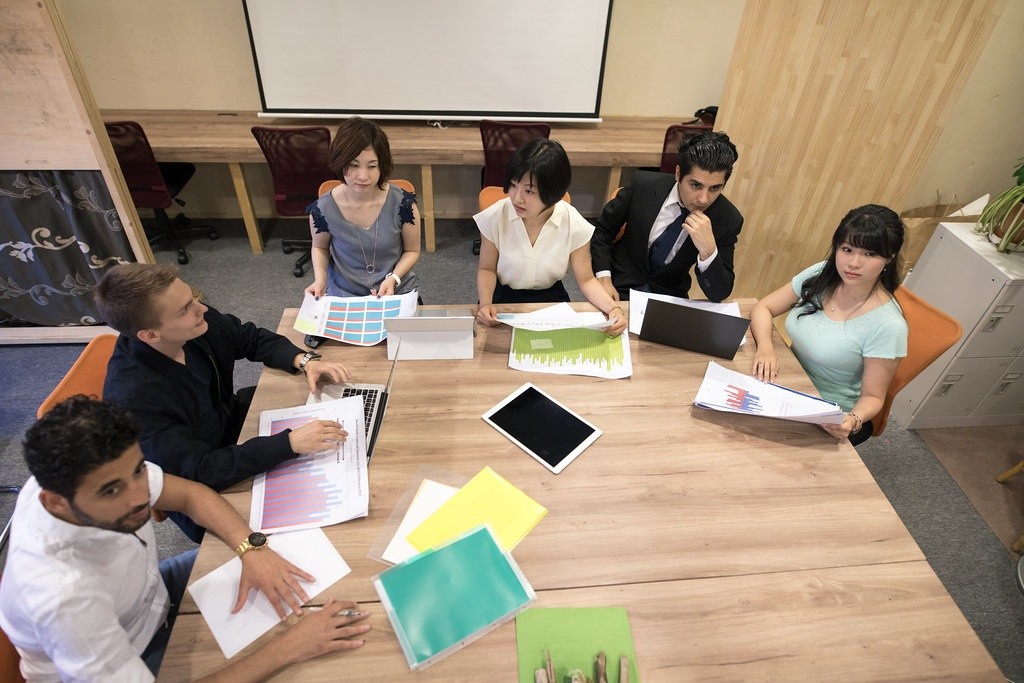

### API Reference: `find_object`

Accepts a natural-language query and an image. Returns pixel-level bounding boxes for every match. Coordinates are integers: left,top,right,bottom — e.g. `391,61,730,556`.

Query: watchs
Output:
386,272,401,288
299,350,320,376
236,532,268,558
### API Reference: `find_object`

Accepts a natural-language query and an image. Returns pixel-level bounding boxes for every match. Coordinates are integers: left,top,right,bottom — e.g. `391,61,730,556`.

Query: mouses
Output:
304,334,327,348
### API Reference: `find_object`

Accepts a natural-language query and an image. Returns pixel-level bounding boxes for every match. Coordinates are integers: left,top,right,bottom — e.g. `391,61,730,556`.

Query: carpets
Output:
917,422,1024,563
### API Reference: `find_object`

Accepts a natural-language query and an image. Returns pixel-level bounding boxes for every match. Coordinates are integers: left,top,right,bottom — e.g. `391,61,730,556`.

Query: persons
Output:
95,262,350,544
749,203,908,440
473,137,627,336
591,131,743,301
0,393,370,683
305,117,421,305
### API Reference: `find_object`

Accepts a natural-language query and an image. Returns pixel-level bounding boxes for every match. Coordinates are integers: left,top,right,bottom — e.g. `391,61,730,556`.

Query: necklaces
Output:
343,184,379,273
831,285,874,310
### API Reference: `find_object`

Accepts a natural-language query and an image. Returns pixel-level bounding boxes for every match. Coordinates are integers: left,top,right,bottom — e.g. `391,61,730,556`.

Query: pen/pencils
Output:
308,606,361,616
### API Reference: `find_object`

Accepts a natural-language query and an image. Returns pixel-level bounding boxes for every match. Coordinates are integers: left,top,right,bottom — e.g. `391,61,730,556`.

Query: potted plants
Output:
974,154,1024,254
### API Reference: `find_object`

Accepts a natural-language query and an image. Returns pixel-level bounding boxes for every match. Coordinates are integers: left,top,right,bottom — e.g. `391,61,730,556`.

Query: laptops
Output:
384,308,477,338
307,337,402,467
638,298,752,361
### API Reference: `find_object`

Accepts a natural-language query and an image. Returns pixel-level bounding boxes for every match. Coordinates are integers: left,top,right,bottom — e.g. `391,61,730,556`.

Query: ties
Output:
648,202,690,274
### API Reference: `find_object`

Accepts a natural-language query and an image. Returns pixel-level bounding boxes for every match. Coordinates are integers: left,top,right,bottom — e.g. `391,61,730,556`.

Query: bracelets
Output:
607,305,623,315
848,412,862,434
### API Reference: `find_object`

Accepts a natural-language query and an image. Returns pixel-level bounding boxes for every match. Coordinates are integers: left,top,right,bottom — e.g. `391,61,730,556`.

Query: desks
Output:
154,298,1015,683
97,108,702,255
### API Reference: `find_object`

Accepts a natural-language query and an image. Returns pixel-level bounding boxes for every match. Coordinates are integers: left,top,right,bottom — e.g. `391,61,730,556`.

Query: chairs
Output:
659,124,717,175
847,283,962,443
477,186,572,212
103,120,220,266
470,120,551,255
36,333,118,422
251,124,340,279
319,178,415,199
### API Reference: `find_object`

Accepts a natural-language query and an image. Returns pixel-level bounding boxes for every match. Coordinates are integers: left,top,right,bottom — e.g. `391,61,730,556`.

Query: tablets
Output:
481,381,602,475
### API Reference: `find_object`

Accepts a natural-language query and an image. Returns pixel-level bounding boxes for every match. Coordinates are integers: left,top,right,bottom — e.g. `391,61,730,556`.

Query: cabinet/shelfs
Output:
890,223,1024,431
